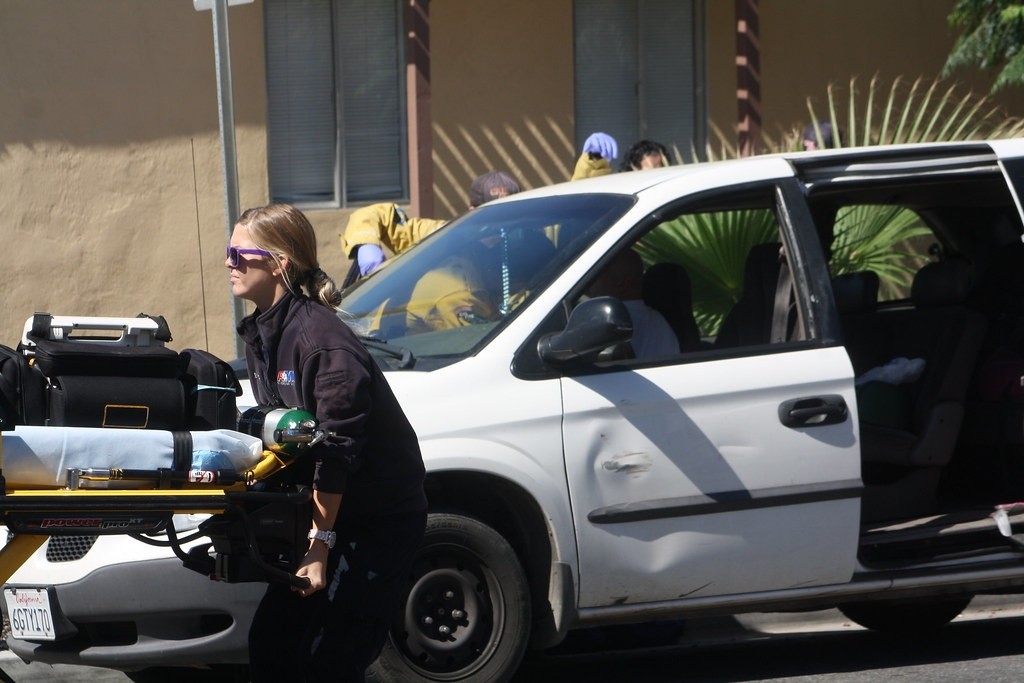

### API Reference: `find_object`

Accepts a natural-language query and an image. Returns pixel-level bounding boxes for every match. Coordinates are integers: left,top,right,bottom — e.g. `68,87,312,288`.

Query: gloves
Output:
357,245,384,276
584,132,618,163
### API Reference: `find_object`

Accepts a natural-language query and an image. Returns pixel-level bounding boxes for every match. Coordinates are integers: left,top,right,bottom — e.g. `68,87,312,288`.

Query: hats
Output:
470,171,521,207
803,120,843,149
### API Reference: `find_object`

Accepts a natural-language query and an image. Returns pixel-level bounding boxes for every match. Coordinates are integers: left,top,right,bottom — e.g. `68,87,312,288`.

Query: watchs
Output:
308,529,336,548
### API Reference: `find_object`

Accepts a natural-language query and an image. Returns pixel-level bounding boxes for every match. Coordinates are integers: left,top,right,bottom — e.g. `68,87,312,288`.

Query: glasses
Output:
227,247,274,268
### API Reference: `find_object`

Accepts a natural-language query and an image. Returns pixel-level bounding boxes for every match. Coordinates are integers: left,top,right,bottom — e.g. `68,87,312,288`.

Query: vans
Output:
227,138,1024,683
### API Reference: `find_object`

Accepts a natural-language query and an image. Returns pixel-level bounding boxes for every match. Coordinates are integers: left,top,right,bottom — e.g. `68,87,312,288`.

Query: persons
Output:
544,133,666,243
585,250,681,363
226,206,427,683
803,121,843,151
342,172,553,337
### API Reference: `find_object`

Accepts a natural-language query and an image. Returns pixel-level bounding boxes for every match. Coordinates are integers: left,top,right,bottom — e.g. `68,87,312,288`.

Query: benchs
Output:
837,258,986,468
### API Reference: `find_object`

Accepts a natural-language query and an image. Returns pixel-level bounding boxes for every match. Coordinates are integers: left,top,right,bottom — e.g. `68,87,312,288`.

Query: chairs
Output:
639,263,702,355
711,242,798,349
